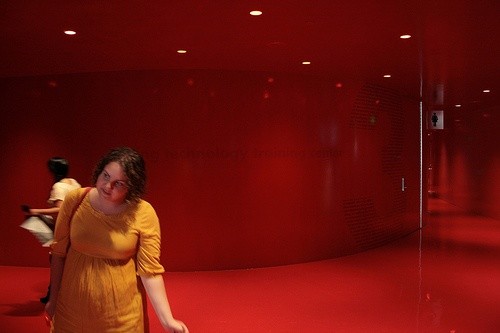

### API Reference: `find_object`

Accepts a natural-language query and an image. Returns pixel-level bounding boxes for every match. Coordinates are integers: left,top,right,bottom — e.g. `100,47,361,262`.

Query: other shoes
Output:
40,288,51,303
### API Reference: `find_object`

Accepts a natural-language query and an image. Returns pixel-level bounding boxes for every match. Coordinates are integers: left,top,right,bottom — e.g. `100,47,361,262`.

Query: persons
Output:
44,148,190,333
24,156,83,303
